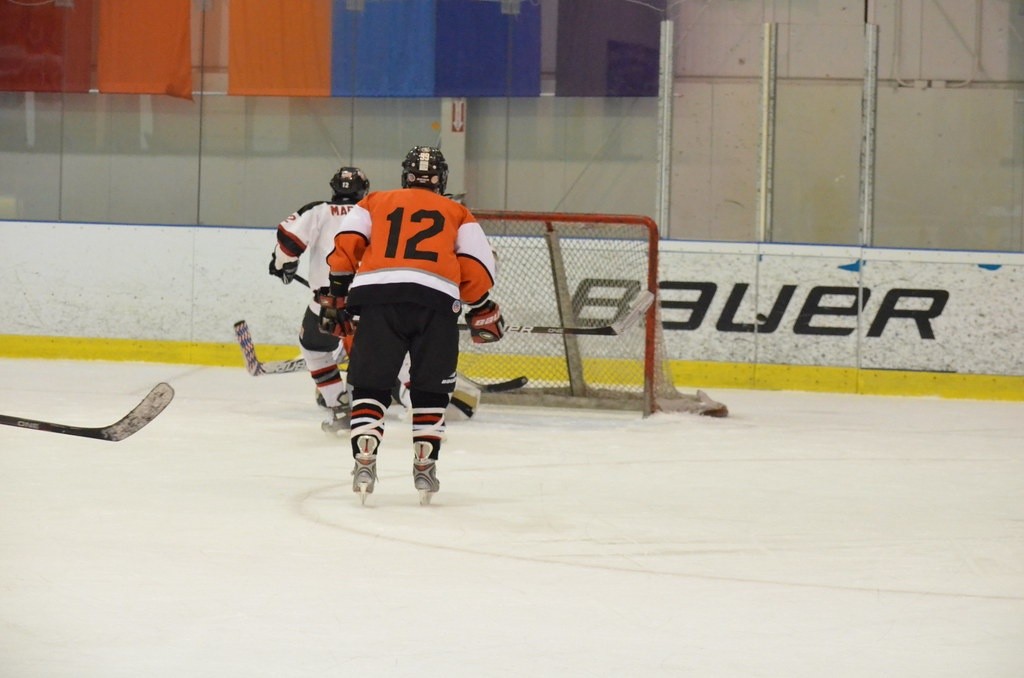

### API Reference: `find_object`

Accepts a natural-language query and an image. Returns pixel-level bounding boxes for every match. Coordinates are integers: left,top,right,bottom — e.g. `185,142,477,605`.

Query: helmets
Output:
402,146,449,194
329,167,369,200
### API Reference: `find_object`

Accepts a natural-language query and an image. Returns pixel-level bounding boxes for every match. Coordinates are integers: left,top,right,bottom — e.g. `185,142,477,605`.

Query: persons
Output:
326,146,506,506
270,166,411,436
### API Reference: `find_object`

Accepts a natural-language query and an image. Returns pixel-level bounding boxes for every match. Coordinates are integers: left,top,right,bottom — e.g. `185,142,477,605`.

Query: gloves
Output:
268,253,300,284
465,301,505,343
317,291,357,338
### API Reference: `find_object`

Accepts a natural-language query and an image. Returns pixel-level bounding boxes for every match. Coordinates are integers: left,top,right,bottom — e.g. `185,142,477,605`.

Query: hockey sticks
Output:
293,274,528,394
234,319,306,377
0,382,175,442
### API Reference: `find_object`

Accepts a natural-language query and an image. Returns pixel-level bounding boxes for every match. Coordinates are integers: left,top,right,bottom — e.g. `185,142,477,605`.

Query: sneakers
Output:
413,461,440,492
352,459,377,493
321,406,351,436
